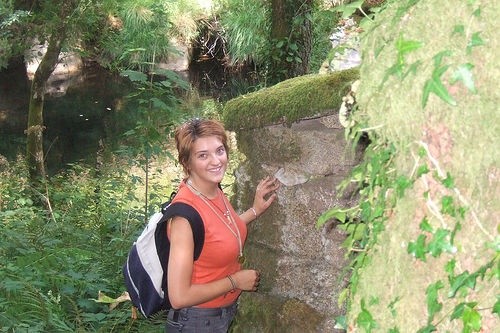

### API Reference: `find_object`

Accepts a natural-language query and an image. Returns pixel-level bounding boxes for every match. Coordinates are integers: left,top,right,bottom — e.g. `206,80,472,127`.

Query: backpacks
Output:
122,192,205,319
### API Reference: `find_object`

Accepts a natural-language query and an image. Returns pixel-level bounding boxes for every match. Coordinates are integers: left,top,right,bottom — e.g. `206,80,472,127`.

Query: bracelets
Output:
250,208,257,219
226,275,235,290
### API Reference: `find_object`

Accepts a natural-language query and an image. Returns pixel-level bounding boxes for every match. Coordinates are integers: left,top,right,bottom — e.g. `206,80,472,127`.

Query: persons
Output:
165,120,279,333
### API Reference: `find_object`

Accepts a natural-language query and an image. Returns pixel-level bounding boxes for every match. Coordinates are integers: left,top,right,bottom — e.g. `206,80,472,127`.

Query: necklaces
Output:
186,179,232,224
183,178,245,264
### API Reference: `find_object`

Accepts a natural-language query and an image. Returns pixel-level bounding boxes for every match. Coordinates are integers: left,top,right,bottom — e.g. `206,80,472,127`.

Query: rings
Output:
256,187,260,191
